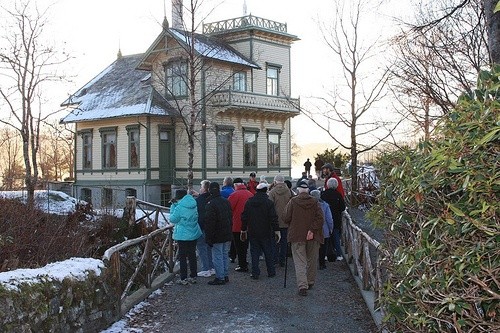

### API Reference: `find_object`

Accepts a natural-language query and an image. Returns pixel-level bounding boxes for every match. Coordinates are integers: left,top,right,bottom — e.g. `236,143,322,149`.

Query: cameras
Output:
168,199,176,204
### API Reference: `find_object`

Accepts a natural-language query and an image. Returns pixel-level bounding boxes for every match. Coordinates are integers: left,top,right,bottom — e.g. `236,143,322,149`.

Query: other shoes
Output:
324,256,328,261
187,277,197,284
178,278,188,286
250,274,257,279
309,284,314,290
209,268,215,275
208,278,226,285
225,276,229,282
228,257,235,263
320,264,326,269
337,256,343,261
299,288,307,296
197,270,211,277
235,266,248,272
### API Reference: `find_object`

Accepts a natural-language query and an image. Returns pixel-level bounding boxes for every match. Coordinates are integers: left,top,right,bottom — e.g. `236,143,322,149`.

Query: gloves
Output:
240,230,247,242
206,238,215,248
274,231,281,243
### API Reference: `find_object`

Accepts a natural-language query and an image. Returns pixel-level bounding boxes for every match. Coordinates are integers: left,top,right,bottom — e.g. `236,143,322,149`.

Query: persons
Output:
169,188,203,287
203,181,235,285
188,179,217,278
303,158,312,176
314,156,324,180
240,182,281,280
281,180,325,297
208,163,348,272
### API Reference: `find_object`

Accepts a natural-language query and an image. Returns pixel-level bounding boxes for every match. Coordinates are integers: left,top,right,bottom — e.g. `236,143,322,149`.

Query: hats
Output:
233,178,244,183
321,163,332,169
175,189,188,200
297,181,309,188
